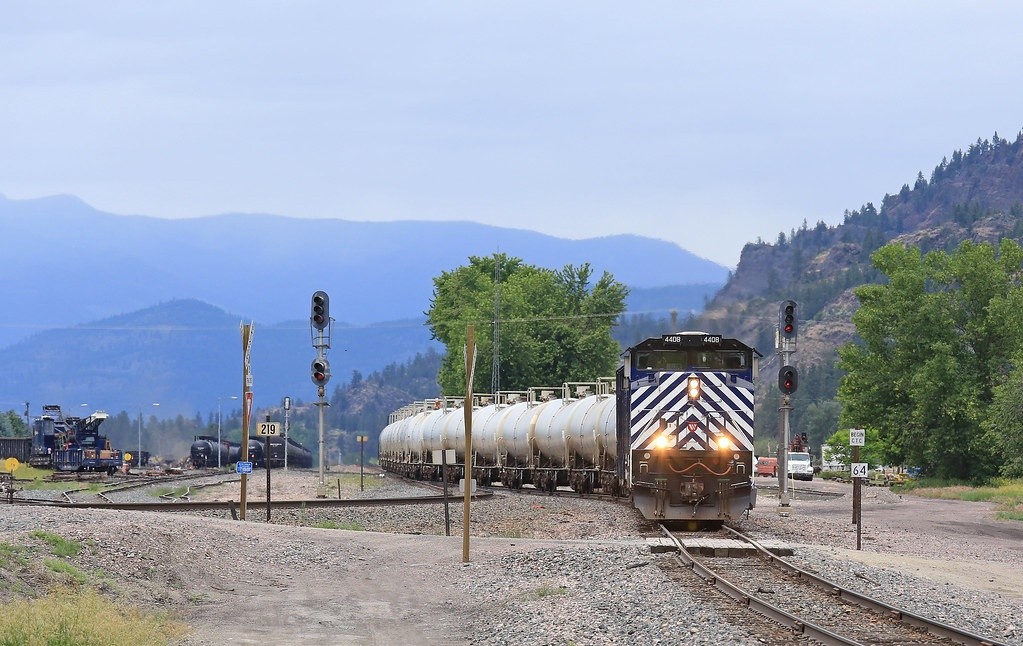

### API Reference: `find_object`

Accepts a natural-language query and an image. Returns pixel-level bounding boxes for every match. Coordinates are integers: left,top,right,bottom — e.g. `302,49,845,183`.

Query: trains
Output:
378,332,764,520
190,433,313,468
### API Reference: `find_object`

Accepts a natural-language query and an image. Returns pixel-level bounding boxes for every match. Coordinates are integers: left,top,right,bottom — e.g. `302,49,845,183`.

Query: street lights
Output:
138,403,159,469
218,397,239,470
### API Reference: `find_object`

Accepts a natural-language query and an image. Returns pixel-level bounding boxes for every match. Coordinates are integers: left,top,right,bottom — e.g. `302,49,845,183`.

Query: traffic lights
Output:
778,299,799,339
311,358,330,386
778,366,798,394
311,291,329,330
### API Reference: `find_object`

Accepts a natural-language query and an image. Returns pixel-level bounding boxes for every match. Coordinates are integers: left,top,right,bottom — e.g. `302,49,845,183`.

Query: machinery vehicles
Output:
28,402,123,475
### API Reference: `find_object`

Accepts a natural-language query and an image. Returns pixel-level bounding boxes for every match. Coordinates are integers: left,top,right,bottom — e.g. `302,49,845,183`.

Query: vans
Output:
788,451,813,481
